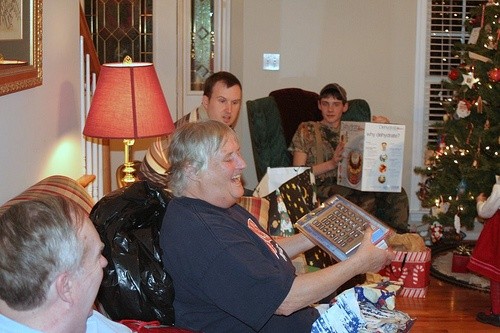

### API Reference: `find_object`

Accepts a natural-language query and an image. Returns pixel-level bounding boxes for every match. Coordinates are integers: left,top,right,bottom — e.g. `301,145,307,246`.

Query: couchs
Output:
0,175,95,220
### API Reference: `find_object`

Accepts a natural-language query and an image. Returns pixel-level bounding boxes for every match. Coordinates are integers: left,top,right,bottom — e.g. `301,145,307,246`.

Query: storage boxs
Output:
337,121,410,193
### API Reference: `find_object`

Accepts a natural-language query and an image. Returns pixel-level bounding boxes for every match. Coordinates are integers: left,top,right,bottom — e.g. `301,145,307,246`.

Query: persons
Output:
288,84,378,211
0,197,139,333
137,72,242,187
466,175,500,326
160,120,394,333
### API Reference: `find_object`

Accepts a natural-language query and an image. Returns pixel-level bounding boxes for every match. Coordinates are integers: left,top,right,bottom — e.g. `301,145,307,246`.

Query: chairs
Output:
247,88,411,268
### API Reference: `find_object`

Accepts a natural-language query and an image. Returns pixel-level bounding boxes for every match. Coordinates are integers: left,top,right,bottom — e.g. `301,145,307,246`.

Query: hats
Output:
320,83,347,102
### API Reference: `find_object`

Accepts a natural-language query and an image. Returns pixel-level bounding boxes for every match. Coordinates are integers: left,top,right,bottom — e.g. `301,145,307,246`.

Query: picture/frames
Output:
0,0,44,96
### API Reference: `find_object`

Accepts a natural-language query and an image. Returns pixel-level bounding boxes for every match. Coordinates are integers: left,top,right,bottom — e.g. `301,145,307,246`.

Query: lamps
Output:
83,56,176,186
